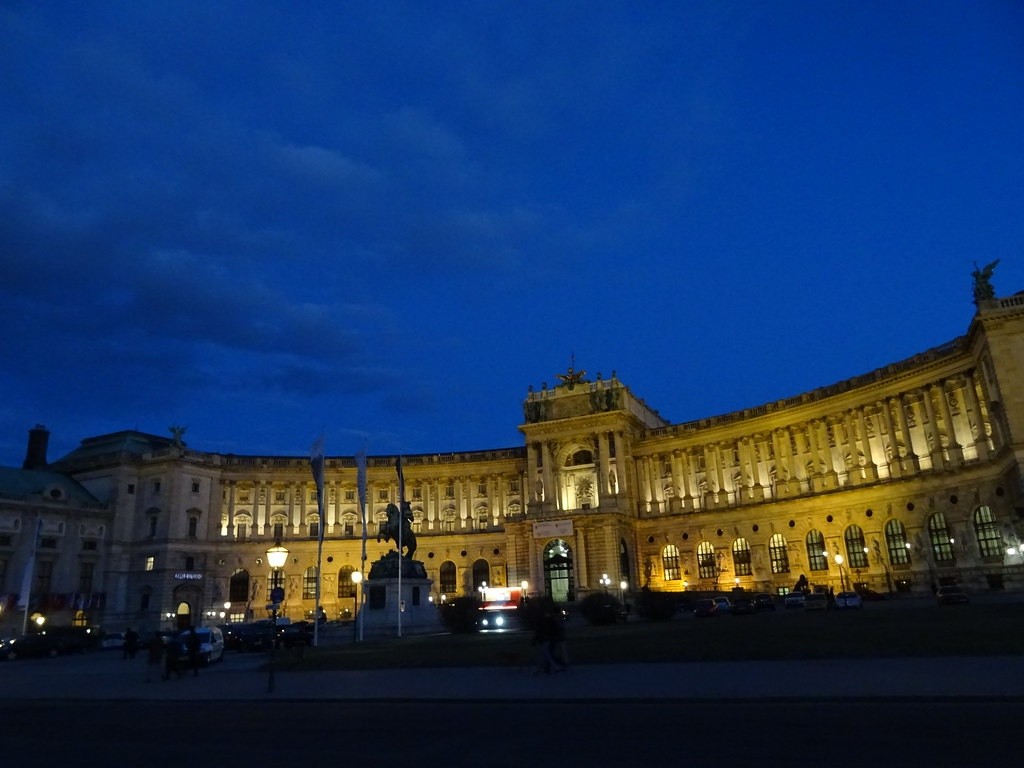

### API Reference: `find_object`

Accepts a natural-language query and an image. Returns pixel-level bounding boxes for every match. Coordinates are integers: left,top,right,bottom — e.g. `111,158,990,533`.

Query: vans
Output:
179,627,224,668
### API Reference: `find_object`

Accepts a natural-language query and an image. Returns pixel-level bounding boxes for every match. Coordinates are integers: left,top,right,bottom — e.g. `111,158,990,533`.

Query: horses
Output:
377,503,417,560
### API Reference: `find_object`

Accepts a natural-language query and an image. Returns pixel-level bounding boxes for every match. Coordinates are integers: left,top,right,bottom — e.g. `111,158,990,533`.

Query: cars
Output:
0,631,94,662
101,632,125,652
218,622,316,653
755,594,771,609
693,598,719,618
732,598,755,615
714,597,731,615
804,593,828,612
785,591,805,610
935,586,970,609
836,593,862,609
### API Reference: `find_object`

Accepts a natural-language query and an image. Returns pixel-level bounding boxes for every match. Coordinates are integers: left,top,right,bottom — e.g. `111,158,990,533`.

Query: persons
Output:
143,630,171,684
183,625,201,677
120,627,140,660
